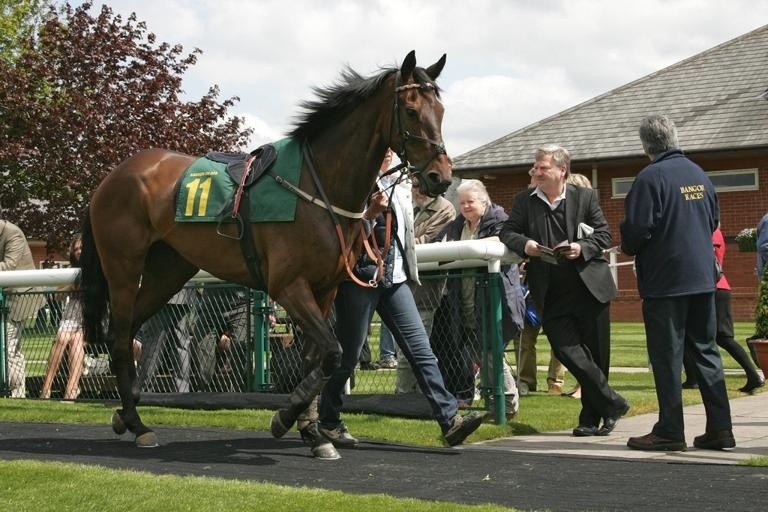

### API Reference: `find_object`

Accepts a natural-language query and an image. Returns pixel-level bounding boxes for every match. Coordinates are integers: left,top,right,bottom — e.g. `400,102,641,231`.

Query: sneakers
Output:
380,356,398,368
444,411,483,446
482,414,496,424
318,420,359,448
506,409,519,420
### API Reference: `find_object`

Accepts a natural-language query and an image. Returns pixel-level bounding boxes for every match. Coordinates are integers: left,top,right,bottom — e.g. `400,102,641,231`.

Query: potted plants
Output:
746,257,768,380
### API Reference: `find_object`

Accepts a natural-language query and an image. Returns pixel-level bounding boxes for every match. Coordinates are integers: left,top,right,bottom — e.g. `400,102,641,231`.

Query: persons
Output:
318,146,482,450
39,235,85,400
682,216,768,392
0,219,48,399
620,114,735,451
395,179,457,395
429,179,526,423
499,147,630,436
473,175,595,398
134,270,400,395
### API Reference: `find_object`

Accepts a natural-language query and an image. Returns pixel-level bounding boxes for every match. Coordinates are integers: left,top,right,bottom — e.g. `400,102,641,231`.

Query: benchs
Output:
55,335,294,397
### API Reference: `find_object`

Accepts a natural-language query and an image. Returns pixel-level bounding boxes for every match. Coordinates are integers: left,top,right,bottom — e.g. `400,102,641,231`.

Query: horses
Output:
78,50,453,460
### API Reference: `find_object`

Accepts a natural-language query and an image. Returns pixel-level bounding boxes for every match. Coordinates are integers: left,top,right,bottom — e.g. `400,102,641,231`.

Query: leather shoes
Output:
627,433,686,451
694,429,735,448
595,400,631,436
741,377,765,392
681,381,699,389
548,383,562,394
359,362,379,369
573,423,599,436
